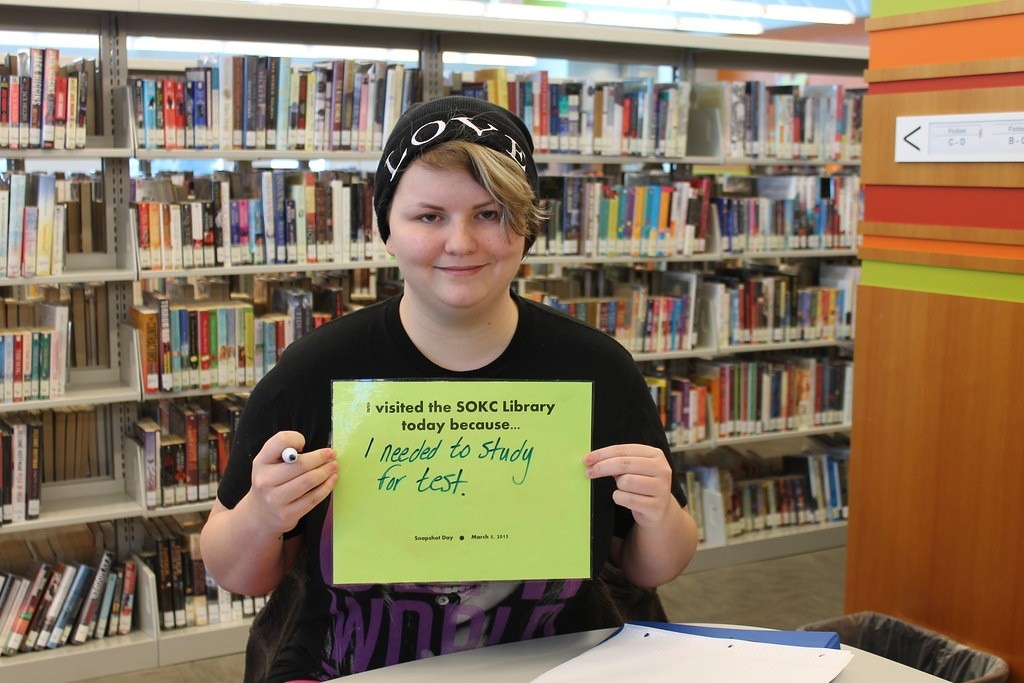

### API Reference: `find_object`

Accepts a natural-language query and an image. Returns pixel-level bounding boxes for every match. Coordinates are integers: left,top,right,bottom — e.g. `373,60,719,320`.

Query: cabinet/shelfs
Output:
712,31,869,575
431,13,712,579
0,2,158,682
115,0,430,669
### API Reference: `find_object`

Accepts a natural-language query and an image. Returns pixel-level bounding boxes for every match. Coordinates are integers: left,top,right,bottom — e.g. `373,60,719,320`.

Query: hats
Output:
374,96,540,252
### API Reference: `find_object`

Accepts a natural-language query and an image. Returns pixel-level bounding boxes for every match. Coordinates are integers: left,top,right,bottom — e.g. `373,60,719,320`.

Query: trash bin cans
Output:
794,610,1010,683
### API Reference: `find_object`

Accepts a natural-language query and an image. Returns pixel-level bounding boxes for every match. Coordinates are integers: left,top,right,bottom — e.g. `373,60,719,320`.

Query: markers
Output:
280,446,299,465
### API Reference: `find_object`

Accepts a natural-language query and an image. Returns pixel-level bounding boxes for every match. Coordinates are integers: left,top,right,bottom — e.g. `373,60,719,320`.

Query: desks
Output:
322,621,948,681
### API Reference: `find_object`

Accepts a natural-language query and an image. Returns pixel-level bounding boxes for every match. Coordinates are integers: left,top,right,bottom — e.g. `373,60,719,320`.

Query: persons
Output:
202,95,699,683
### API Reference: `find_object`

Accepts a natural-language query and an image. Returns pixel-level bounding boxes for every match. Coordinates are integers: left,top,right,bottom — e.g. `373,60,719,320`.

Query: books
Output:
1,428,848,668
0,163,867,279
0,258,861,407
0,347,854,531
0,39,876,158
